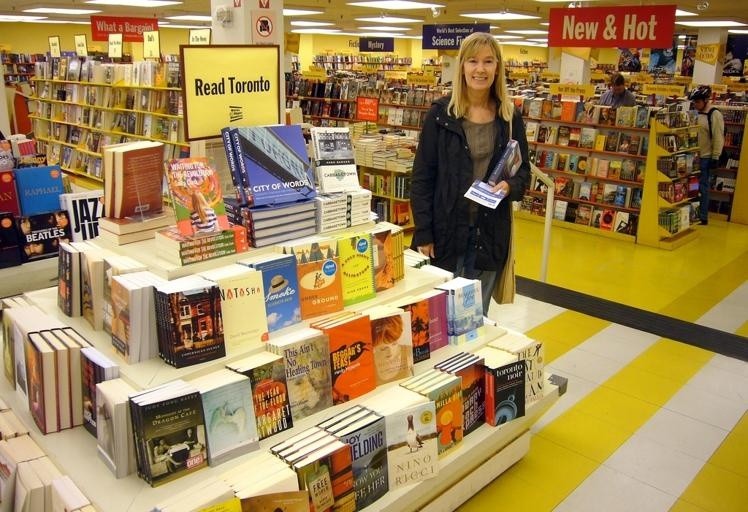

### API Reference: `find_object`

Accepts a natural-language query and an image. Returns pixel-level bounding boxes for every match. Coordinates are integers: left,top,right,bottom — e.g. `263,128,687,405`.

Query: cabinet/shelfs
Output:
422,59,443,83
285,72,448,143
637,102,748,222
508,96,650,244
302,144,417,232
0,65,33,141
0,52,133,114
313,52,412,78
27,57,190,206
637,119,704,251
589,64,615,83
503,61,548,87
0,182,91,298
1,221,567,511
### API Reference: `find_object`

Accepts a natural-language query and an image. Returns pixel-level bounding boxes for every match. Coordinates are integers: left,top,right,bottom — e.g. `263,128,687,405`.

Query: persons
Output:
183,428,197,451
154,438,182,473
599,74,636,108
17,210,69,258
371,316,414,385
687,86,724,224
412,299,428,320
410,33,532,318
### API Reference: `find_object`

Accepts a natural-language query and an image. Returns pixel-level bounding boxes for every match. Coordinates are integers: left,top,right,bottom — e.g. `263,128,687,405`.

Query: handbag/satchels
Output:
490,103,518,306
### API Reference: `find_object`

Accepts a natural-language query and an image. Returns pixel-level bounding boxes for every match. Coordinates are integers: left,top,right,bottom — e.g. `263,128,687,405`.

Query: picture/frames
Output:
178,44,280,141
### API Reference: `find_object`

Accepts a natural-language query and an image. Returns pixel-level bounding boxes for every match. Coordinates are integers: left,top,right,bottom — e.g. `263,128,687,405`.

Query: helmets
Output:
687,85,712,101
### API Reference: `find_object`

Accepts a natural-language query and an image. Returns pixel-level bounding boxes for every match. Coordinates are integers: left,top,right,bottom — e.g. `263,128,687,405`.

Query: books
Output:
0,133,105,269
27,62,191,181
0,397,98,512
357,351,488,490
344,121,415,226
98,125,373,268
128,350,295,488
153,222,406,369
55,241,166,365
656,104,690,235
0,294,135,480
266,277,486,422
287,56,441,138
154,331,545,510
510,98,649,235
152,406,389,512
